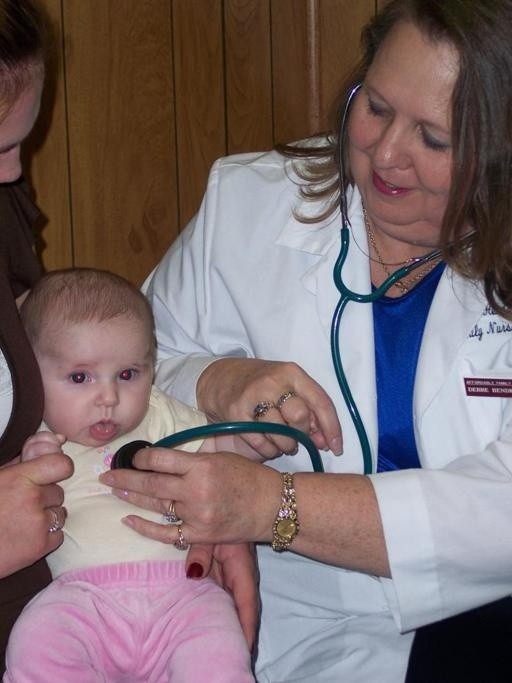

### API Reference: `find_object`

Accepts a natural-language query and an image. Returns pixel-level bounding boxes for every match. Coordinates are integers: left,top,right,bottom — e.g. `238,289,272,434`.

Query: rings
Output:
47,505,65,533
252,400,275,420
276,389,296,410
175,523,189,551
165,498,178,522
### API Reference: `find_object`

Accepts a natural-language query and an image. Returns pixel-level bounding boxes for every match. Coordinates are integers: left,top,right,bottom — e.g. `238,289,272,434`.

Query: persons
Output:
0,266,264,681
95,0,511,682
0,2,264,682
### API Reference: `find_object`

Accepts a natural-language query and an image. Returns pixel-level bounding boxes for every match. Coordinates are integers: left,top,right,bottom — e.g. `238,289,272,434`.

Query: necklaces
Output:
361,198,448,295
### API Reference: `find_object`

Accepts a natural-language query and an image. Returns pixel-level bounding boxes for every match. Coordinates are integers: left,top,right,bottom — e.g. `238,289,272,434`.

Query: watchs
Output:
270,469,301,553
